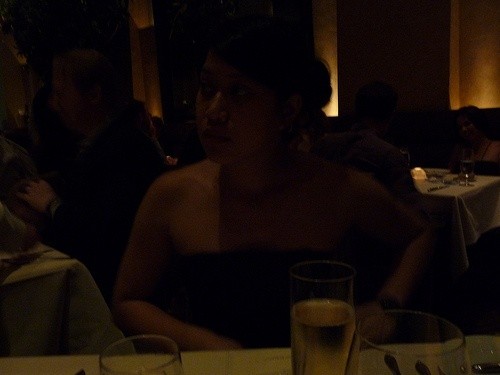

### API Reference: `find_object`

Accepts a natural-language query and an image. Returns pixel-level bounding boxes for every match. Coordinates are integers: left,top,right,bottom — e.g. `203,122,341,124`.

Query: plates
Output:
437,335,500,375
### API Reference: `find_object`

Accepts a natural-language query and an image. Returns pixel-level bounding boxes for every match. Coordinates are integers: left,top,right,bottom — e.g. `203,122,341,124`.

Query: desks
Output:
0,335,500,375
416,173,500,270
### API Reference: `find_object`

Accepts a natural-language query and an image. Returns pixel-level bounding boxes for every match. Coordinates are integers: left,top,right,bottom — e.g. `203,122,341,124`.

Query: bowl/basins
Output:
424,168,450,177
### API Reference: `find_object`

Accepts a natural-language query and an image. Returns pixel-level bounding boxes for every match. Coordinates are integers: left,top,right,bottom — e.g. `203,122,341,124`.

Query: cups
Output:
343,310,471,375
457,148,475,186
98,334,185,375
288,261,359,375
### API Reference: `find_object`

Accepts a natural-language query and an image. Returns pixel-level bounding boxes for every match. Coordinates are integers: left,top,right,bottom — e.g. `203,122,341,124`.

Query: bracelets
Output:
378,299,400,314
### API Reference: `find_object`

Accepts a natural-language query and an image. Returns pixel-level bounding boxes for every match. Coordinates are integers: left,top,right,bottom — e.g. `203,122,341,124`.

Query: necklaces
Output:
480,141,493,161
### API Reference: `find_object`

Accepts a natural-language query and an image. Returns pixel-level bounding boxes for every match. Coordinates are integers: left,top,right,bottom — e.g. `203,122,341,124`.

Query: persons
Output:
0,49,164,358
283,57,453,272
110,13,437,351
452,102,500,177
